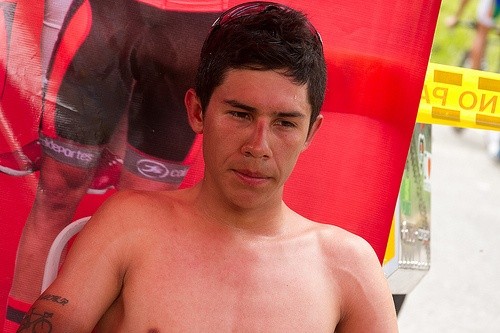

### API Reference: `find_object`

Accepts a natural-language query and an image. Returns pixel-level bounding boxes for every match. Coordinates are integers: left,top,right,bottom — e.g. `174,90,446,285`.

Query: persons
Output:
6,1,227,332
15,7,399,333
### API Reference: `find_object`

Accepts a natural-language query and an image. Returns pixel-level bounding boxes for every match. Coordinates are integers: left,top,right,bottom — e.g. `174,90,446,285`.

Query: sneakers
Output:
86,148,124,195
0,140,44,177
